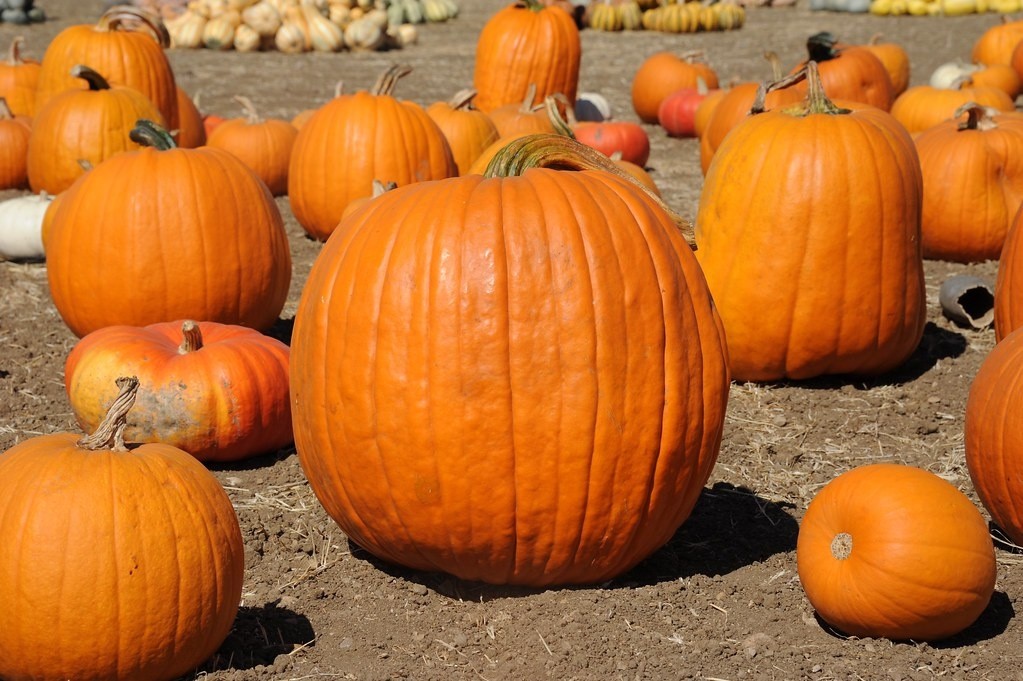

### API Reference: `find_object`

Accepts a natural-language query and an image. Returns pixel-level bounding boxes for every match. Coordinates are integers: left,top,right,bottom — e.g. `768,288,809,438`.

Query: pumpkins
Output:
796,465,996,644
0,0,1023,584
0,376,247,681
